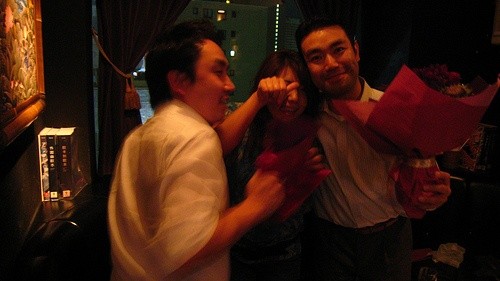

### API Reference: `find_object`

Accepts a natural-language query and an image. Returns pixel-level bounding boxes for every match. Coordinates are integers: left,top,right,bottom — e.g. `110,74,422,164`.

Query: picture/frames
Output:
0,0,48,150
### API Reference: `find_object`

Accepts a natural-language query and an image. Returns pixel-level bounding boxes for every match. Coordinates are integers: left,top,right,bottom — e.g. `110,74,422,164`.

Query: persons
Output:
295,14,451,281
212,49,330,281
106,18,289,281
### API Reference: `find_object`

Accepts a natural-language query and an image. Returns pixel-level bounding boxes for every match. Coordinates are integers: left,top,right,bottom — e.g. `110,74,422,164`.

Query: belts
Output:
355,216,400,236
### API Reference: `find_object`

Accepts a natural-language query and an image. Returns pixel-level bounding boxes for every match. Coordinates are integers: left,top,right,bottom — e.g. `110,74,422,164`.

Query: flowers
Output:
332,64,500,219
